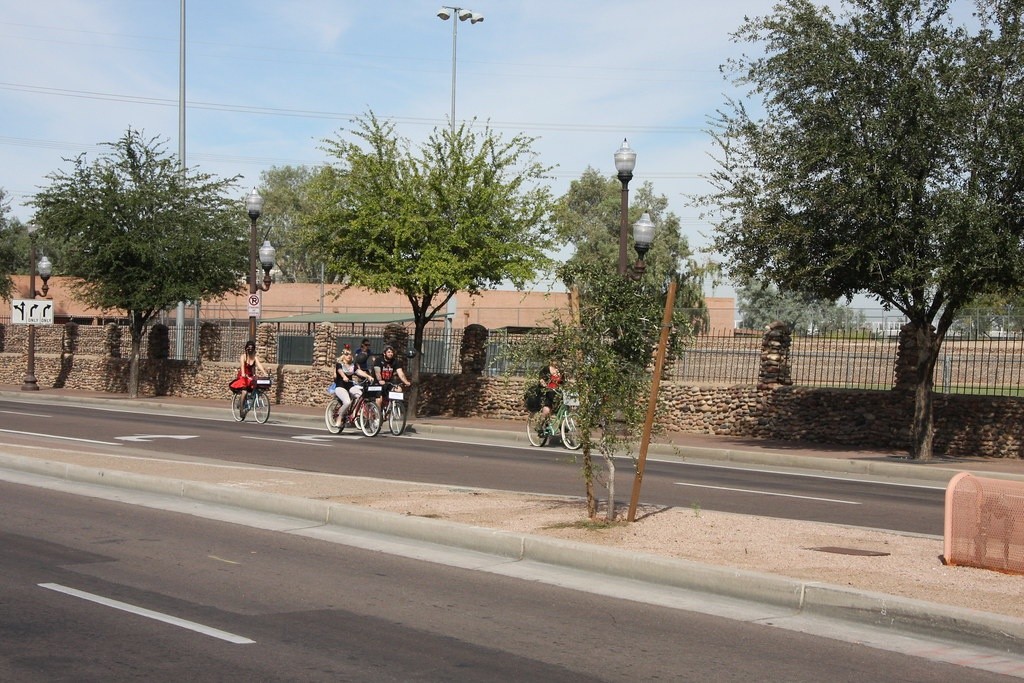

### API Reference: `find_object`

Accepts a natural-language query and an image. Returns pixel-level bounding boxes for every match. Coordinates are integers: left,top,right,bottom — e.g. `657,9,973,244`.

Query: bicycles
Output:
525,381,585,450
229,368,272,424
327,392,369,429
322,377,384,438
351,377,412,435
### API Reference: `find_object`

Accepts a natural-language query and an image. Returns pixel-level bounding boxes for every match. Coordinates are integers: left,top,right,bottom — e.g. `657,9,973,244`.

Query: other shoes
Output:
240,407,245,418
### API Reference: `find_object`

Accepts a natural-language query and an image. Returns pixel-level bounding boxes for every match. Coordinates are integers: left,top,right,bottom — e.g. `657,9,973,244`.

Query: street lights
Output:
436,5,484,183
16,220,54,393
230,186,278,342
611,136,656,286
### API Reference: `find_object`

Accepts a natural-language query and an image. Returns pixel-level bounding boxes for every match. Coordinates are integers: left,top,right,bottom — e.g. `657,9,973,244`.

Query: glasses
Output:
364,344,370,346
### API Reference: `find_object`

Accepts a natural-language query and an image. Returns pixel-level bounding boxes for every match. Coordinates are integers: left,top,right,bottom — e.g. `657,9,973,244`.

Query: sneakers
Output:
336,417,342,428
558,424,570,431
534,424,544,438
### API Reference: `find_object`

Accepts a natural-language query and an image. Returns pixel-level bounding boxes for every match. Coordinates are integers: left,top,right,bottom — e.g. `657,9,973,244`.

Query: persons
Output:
375,345,412,429
334,357,362,423
327,344,375,429
230,342,270,420
356,338,372,387
364,356,380,430
537,355,571,437
